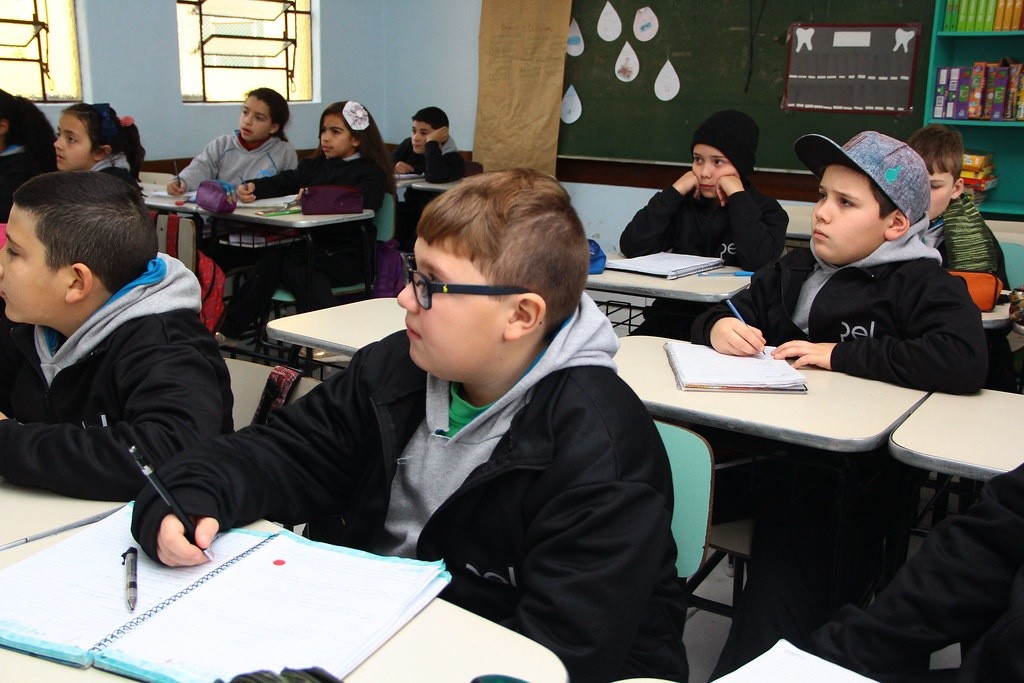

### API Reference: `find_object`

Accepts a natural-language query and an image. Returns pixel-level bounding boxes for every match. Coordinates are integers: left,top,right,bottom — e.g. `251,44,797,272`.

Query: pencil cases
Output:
303,184,364,213
194,178,237,215
950,270,1001,312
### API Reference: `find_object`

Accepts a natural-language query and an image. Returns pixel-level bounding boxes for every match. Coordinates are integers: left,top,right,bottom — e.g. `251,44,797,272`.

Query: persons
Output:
904,124,1015,395
217,100,397,362
619,110,789,342
0,88,58,224
705,130,987,683
0,170,236,503
168,87,298,272
54,102,146,203
130,168,690,683
392,105,467,270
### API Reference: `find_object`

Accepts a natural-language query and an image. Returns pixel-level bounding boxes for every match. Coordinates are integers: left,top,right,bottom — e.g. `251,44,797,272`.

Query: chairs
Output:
138,172,175,184
990,231,1024,290
251,194,395,363
220,355,321,431
648,415,755,627
155,214,195,273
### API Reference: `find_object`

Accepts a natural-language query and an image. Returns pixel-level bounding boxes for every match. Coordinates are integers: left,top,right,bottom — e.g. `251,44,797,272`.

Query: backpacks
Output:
149,210,225,334
366,239,406,300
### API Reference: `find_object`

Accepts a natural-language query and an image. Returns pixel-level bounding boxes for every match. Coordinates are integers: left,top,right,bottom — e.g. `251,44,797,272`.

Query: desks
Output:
5,205,1024,683
137,182,459,367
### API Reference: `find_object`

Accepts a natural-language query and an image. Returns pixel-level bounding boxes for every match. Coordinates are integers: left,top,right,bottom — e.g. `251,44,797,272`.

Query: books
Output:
603,252,725,281
150,191,198,204
0,499,452,683
236,194,299,210
663,339,809,395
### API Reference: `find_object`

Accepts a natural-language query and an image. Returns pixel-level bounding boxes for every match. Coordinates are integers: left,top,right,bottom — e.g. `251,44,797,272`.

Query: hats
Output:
794,131,932,224
689,110,758,190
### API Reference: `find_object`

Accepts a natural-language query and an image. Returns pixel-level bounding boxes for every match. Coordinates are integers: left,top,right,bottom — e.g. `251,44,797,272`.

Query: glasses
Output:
400,252,544,326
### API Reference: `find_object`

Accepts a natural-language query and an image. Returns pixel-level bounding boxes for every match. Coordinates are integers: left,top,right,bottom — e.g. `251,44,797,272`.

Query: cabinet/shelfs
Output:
923,0,1024,215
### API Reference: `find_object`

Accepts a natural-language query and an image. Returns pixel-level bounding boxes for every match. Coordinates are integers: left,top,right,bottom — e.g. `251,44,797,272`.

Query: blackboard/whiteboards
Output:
558,0,937,204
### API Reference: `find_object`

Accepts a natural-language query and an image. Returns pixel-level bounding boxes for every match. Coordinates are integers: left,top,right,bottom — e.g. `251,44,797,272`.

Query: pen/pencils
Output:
696,270,755,282
726,297,769,357
173,159,180,188
239,173,253,195
129,443,216,565
121,545,139,612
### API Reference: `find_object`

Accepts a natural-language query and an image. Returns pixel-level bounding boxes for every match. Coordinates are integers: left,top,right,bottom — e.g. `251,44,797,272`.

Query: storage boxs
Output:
960,149,1000,191
933,57,1024,121
